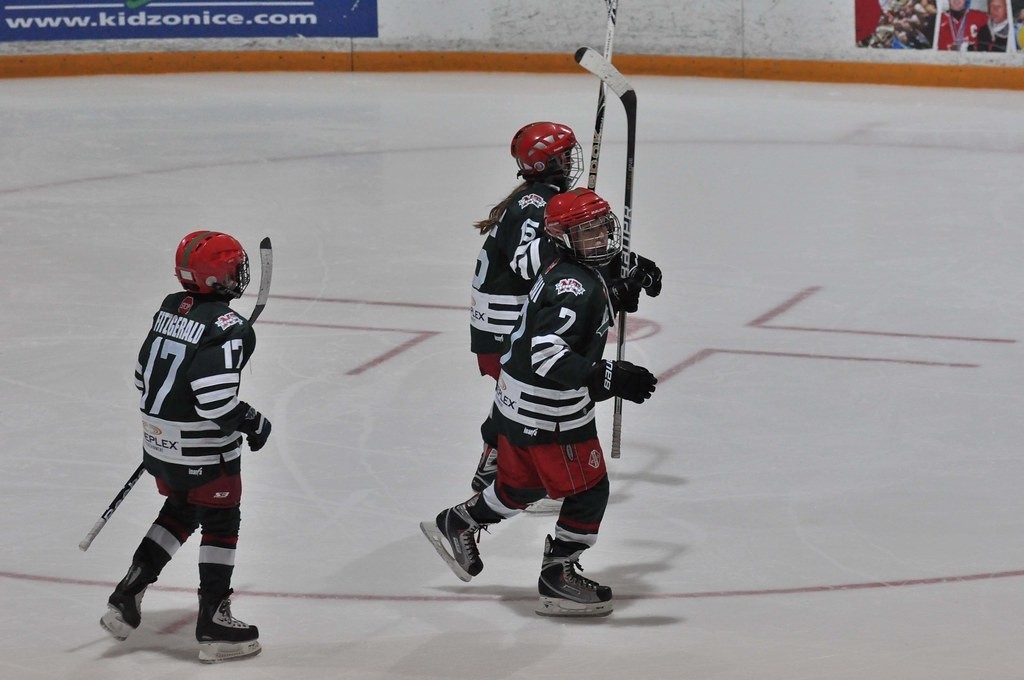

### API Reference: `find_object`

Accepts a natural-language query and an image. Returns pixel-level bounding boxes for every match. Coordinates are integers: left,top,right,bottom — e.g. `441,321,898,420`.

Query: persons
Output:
420,186,657,616
99,231,273,665
1013,8,1024,52
974,0,1009,52
855,0,937,49
939,0,989,52
471,122,662,515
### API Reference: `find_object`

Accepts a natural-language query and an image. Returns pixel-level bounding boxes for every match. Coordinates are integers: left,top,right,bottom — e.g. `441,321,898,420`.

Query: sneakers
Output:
534,533,614,618
100,561,150,643
467,443,500,493
194,587,263,664
419,490,503,583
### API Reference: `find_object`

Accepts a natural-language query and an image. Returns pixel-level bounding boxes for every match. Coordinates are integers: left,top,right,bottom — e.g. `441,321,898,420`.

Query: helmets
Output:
510,121,584,189
543,186,622,264
175,230,251,300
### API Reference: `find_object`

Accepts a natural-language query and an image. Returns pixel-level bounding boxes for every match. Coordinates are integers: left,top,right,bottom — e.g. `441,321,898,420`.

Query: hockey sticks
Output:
586,0,619,192
574,46,638,459
79,237,273,552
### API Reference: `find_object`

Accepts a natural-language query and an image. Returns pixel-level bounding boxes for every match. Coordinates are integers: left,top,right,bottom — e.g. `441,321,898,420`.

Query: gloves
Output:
604,276,643,314
235,403,272,452
629,251,662,297
588,359,657,403
